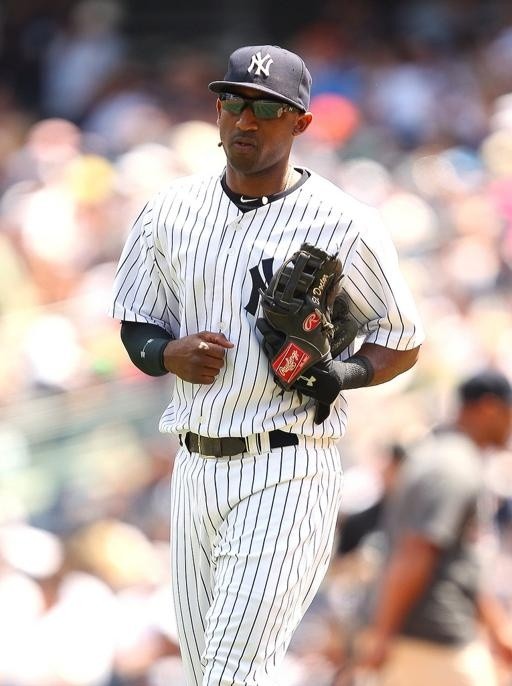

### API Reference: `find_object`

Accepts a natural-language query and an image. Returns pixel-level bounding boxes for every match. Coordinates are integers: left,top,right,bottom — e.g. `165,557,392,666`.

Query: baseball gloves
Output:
259,242,359,391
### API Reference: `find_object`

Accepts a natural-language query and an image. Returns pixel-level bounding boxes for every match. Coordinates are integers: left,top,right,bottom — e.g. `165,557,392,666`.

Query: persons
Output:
111,46,427,686
319,366,511,686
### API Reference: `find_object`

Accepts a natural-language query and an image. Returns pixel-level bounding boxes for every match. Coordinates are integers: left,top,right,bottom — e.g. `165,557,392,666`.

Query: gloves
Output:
256,317,374,425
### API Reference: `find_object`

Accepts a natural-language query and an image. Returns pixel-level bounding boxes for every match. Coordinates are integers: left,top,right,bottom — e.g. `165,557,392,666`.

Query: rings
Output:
197,340,209,355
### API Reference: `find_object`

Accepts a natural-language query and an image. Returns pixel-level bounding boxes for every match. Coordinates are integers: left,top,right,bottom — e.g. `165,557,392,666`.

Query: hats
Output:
206,42,314,116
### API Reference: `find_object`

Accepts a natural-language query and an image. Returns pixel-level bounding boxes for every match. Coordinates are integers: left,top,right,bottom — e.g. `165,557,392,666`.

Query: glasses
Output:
216,89,301,122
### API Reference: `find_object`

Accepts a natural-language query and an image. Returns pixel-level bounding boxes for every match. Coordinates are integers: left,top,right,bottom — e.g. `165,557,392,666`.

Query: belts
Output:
177,427,299,457
